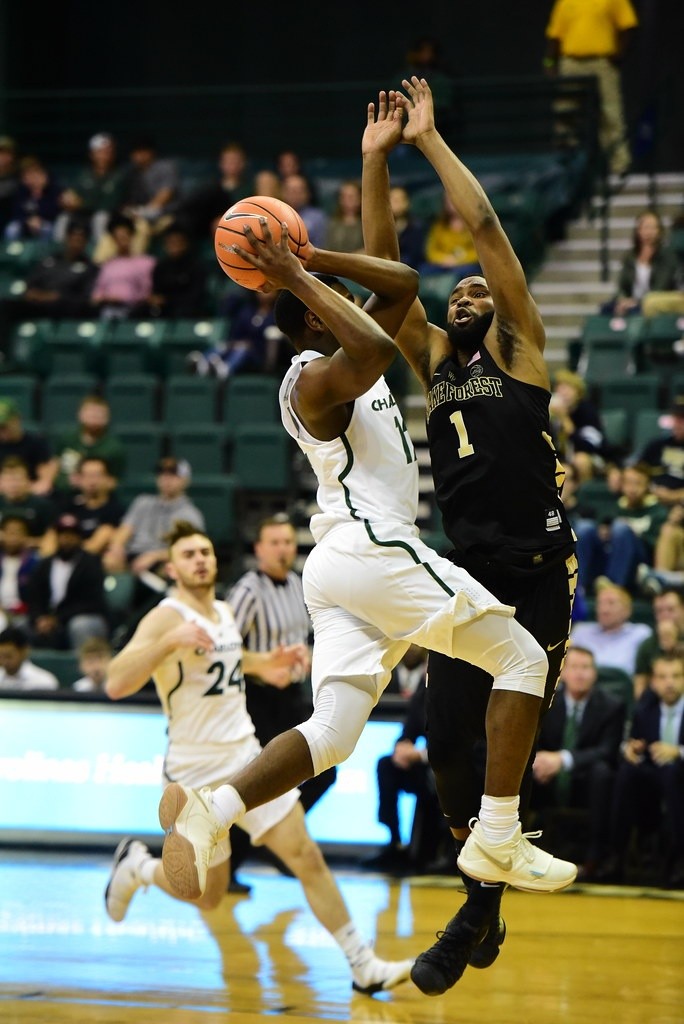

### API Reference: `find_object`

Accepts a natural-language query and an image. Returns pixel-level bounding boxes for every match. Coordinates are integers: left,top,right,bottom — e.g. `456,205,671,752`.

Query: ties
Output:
559,705,581,791
663,712,676,768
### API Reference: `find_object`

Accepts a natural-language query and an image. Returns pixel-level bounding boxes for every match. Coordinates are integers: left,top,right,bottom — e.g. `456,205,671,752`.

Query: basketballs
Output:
214,196,309,292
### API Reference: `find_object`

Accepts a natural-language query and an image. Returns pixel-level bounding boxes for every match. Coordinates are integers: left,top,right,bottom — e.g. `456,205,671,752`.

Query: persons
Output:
0,0,683,998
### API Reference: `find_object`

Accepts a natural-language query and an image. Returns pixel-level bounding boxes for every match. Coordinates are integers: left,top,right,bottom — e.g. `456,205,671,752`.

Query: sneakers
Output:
104,837,152,922
158,781,228,901
352,959,416,997
410,907,506,997
455,817,579,895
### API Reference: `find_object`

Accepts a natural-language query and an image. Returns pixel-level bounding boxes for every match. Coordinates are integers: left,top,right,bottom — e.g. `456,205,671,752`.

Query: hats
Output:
155,457,190,481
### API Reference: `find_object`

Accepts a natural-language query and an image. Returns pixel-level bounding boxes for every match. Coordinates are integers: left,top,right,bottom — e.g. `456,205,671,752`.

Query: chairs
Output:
0,151,684,802
